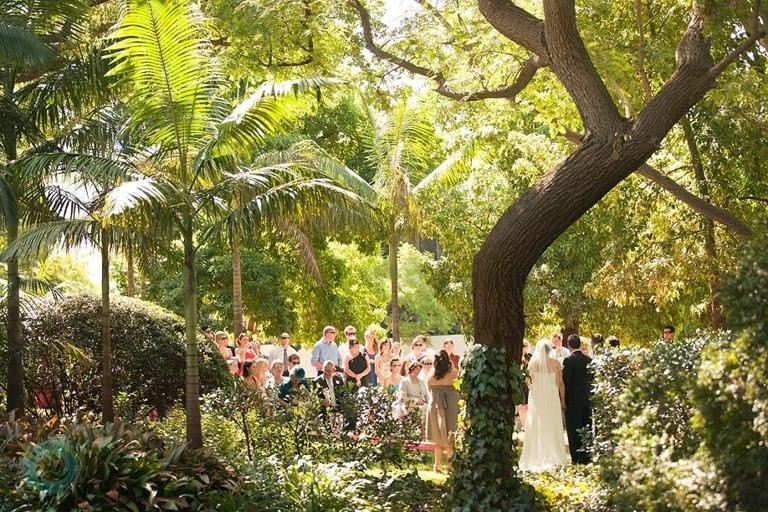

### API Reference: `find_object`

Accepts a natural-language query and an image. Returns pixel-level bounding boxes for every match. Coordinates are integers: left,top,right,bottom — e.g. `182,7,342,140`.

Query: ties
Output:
328,381,336,407
284,349,288,371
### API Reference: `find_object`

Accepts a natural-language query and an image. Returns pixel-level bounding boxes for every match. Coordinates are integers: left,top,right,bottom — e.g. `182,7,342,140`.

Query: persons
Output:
662,325,676,342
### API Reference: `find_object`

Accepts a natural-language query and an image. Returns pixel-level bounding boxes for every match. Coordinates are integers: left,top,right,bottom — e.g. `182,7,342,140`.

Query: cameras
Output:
248,342,254,348
334,365,343,372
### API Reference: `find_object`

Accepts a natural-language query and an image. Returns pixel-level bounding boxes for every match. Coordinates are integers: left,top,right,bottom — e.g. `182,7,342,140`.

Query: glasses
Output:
427,363,432,365
348,333,356,336
282,337,290,339
394,365,401,367
221,337,230,340
414,344,422,346
293,362,300,365
